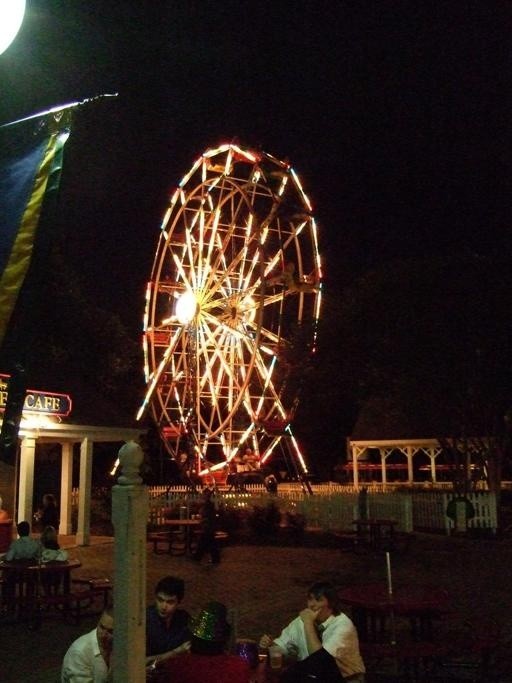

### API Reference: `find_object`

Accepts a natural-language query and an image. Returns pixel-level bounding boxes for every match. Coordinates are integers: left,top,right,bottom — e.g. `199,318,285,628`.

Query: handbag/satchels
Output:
35,548,68,562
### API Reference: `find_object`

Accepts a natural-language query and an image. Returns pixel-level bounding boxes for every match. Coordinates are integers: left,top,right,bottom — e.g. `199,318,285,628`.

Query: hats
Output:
187,601,228,642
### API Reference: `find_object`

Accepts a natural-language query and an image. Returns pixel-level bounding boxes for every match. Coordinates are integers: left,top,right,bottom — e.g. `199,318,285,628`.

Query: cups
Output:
268,646,283,668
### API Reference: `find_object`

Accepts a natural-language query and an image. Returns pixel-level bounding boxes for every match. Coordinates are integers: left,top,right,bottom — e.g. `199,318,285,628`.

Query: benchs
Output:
147,514,231,560
1,547,115,632
296,516,512,681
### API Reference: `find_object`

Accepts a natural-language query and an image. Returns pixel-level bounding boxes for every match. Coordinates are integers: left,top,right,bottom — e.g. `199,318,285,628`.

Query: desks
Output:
145,633,301,683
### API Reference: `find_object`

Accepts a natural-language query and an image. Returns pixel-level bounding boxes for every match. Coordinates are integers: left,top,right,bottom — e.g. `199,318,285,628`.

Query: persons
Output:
260,580,366,683
191,490,220,564
60,606,152,683
38,525,60,594
5,519,39,602
41,493,59,530
146,577,194,664
